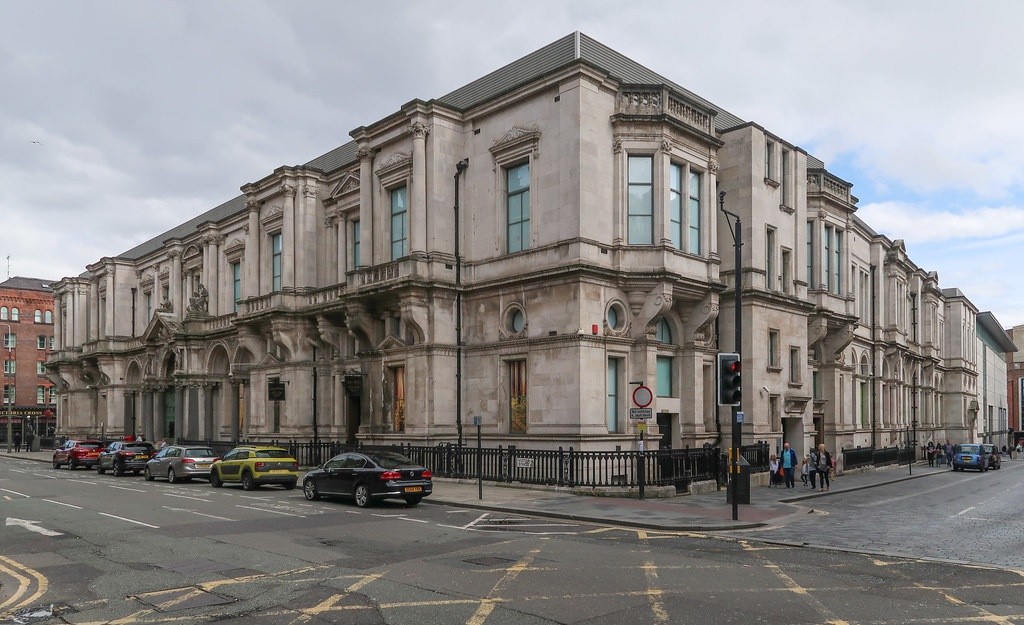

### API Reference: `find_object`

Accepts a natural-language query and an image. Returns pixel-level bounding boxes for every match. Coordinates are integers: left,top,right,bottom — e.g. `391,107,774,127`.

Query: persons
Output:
161,437,168,449
927,440,953,467
155,295,169,312
195,283,208,311
136,434,144,441
25,431,34,452
13,432,22,452
768,442,835,492
1002,442,1024,459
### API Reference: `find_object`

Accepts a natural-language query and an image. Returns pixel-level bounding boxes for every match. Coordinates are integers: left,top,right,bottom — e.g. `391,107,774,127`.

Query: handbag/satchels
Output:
779,467,784,476
820,461,827,472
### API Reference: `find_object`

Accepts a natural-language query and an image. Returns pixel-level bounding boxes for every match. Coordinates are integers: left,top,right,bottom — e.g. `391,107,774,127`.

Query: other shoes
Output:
811,486,816,490
818,487,823,492
827,487,830,491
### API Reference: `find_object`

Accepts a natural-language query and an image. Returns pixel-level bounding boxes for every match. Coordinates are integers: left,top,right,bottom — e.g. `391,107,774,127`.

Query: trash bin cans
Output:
727,454,750,504
439,441,450,473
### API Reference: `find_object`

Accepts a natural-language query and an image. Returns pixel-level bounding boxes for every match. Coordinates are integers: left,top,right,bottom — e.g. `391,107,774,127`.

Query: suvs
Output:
53,439,107,470
97,441,158,476
210,445,299,491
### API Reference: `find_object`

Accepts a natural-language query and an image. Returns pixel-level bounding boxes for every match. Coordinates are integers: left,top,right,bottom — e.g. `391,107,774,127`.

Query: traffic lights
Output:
719,354,743,405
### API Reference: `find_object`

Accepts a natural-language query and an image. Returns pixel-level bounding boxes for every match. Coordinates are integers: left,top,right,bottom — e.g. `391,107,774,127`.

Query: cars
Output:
953,443,990,472
303,450,435,509
144,445,221,483
983,445,1003,470
